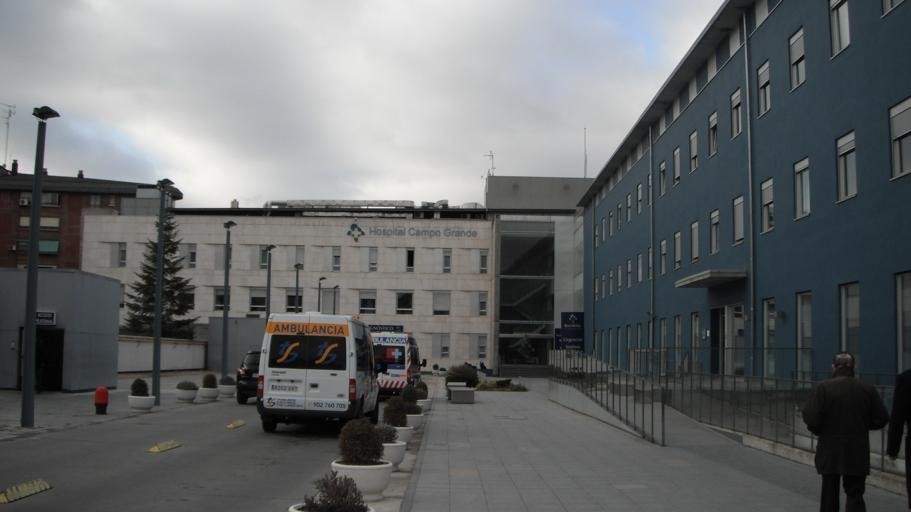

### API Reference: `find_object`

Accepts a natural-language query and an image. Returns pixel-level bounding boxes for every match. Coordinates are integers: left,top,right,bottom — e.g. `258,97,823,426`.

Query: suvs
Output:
236,350,262,405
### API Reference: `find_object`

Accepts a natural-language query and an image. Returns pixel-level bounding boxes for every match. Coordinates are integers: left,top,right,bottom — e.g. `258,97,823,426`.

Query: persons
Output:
885,367,911,512
465,359,486,372
801,352,889,512
432,364,446,371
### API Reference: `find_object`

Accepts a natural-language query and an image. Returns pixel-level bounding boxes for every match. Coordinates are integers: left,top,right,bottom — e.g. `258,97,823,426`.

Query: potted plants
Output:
128,373,237,413
288,380,432,511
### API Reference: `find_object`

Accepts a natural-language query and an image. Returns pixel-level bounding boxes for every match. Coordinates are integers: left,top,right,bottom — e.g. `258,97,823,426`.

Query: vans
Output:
370,331,427,397
256,311,381,434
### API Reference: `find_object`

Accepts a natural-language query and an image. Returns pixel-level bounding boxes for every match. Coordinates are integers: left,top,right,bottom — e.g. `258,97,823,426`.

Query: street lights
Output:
265,244,340,326
220,220,238,379
151,178,184,406
20,105,62,429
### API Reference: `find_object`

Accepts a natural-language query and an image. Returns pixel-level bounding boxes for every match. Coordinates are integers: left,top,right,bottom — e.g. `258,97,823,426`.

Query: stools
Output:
448,385,475,404
